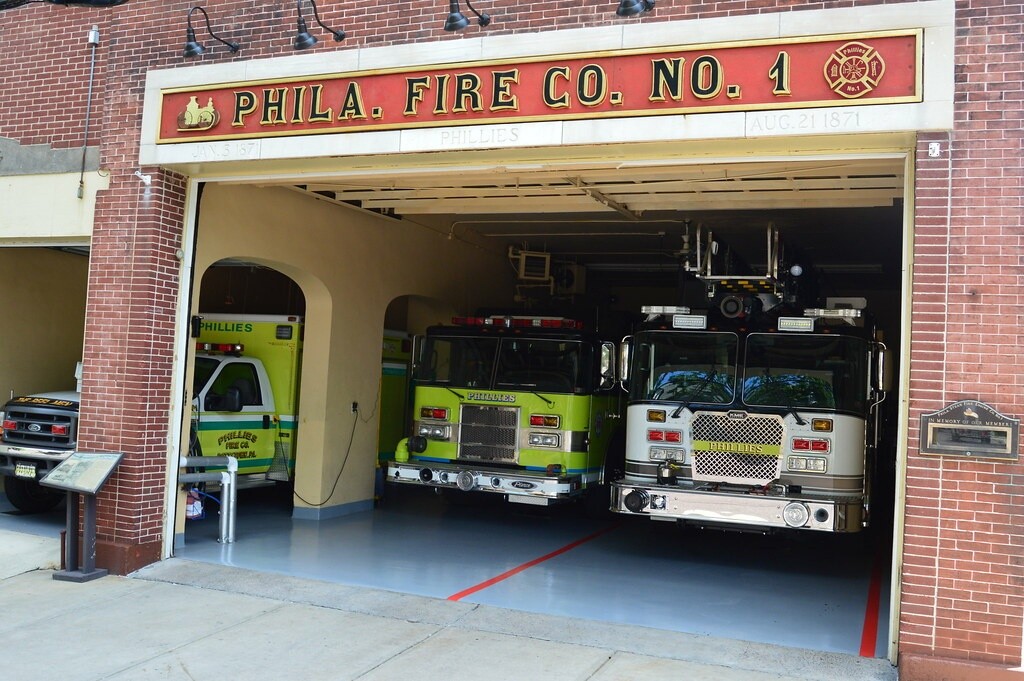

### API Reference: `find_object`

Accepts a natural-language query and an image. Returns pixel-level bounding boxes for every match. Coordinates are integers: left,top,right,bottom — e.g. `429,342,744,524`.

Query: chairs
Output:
232,378,256,406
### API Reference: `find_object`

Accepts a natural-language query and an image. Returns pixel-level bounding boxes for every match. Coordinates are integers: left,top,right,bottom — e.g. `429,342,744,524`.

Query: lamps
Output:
294,0,345,50
444,0,490,32
183,7,240,58
616,0,655,17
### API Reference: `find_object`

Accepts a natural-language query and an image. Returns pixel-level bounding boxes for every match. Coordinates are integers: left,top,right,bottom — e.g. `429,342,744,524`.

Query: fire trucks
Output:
612,219,896,538
380,314,622,508
0,311,411,517
